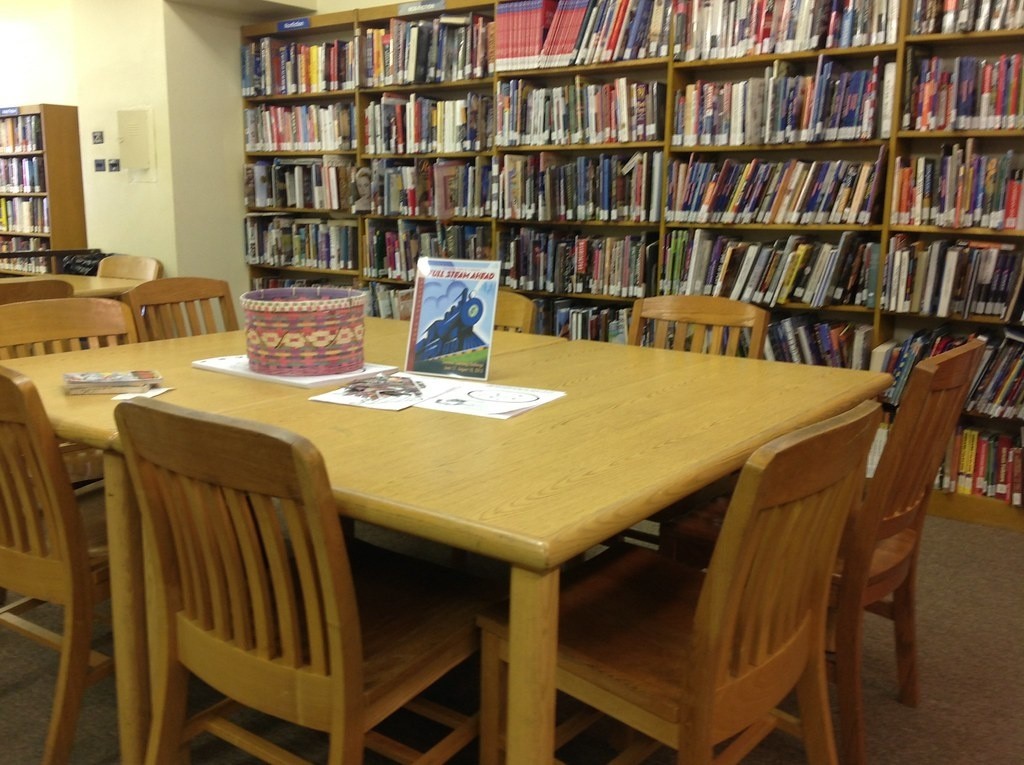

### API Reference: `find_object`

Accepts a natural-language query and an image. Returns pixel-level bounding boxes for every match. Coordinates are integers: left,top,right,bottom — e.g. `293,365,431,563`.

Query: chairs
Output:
0,361,107,765
468,400,883,765
97,255,162,318
629,294,771,360
123,275,242,342
0,297,138,362
112,398,478,765
697,338,989,765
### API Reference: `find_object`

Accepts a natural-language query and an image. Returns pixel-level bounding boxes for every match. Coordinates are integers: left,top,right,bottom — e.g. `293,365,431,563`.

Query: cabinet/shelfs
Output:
0,103,88,277
237,0,1024,535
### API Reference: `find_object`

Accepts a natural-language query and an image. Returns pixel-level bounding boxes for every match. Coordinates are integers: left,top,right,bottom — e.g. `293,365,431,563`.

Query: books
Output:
63,370,164,394
239,0,1024,508
0,114,51,274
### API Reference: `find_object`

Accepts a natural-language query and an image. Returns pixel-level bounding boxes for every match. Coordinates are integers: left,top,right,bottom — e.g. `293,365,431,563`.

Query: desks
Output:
0,274,148,300
0,318,893,765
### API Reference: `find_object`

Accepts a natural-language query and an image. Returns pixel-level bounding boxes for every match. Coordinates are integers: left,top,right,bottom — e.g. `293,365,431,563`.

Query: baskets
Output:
238,284,366,378
